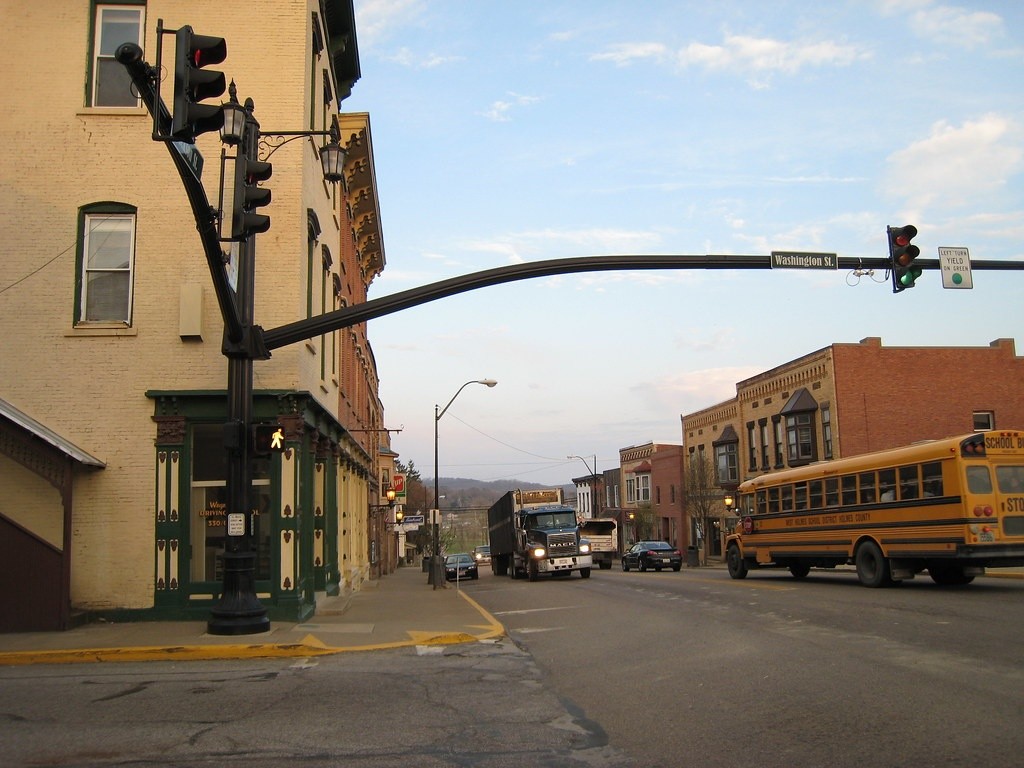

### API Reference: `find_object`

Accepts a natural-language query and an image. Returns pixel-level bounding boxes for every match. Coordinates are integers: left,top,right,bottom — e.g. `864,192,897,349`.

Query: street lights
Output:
567,455,598,519
433,380,498,556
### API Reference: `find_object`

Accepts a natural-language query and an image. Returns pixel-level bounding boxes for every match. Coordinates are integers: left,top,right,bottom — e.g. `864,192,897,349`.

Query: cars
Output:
621,541,682,573
442,554,478,581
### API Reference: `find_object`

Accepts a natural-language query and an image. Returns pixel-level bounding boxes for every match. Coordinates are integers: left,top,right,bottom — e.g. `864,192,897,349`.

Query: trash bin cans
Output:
422,556,431,572
687,545,699,568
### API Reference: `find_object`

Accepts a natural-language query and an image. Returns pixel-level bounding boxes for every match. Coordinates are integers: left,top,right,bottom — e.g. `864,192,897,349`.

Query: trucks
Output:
578,518,619,569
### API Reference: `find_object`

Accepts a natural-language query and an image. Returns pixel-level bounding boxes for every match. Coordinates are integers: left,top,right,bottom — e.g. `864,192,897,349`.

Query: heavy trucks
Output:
488,487,594,582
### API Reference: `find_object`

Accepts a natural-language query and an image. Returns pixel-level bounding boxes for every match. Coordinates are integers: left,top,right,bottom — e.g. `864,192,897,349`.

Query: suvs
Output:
470,545,493,565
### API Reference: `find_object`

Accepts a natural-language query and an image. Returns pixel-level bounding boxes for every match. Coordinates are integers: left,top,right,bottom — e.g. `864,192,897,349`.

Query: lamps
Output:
384,507,403,533
724,496,736,512
369,481,396,520
629,510,635,525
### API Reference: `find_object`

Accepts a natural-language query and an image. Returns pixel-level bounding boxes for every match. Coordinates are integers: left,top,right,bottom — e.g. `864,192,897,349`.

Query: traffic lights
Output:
171,24,228,143
230,152,273,238
886,224,924,293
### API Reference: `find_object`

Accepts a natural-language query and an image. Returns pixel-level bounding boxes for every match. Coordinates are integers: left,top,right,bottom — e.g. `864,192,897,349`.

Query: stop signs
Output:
743,517,753,533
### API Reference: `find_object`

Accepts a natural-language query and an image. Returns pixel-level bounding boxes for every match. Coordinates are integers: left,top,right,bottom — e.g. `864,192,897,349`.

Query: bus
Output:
722,429,1024,589
